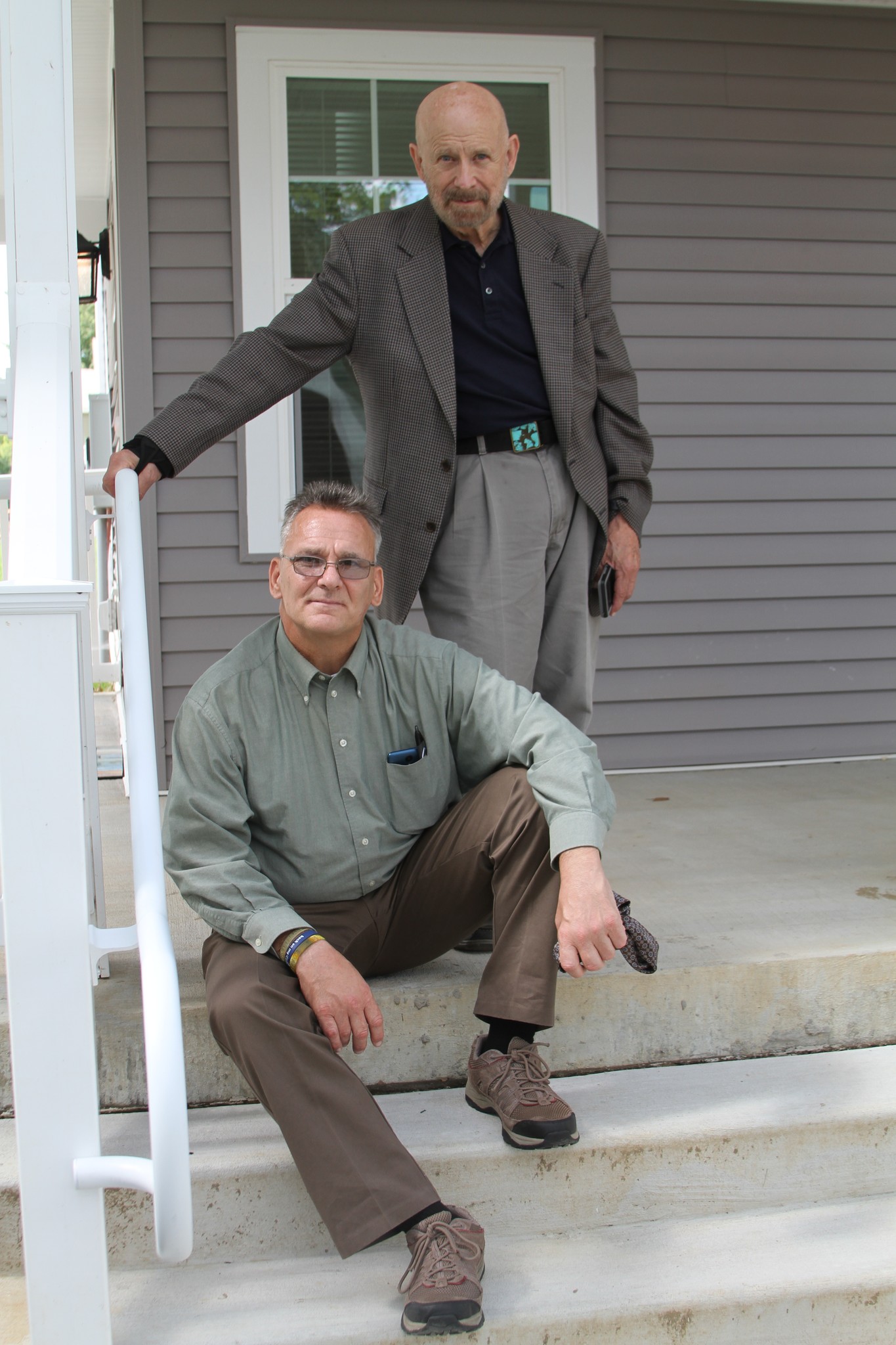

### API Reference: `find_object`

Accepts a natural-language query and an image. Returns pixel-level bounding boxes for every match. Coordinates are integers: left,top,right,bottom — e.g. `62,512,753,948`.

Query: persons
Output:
102,82,654,954
162,481,632,1339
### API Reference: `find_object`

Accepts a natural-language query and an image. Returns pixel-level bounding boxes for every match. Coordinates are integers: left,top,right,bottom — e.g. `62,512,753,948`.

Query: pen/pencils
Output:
415,725,427,759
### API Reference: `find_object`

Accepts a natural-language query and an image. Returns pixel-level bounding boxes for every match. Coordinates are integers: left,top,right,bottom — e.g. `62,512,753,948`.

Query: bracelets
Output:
279,927,327,974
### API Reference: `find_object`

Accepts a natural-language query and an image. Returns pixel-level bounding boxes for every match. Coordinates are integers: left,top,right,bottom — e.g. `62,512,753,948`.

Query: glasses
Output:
280,554,376,581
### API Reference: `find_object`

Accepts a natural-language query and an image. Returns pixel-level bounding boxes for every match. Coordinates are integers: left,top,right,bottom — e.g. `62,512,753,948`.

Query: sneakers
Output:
398,1203,485,1336
465,1033,580,1151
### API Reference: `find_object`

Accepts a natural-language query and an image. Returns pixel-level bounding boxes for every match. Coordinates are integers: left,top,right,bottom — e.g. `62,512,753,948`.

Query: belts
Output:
456,419,557,456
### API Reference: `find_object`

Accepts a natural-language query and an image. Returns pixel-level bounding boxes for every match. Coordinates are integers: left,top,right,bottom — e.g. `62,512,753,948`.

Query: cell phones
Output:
388,748,419,765
597,564,615,618
